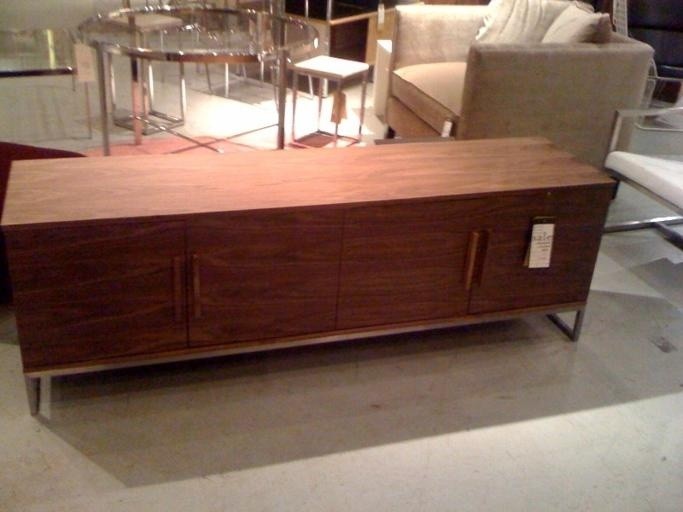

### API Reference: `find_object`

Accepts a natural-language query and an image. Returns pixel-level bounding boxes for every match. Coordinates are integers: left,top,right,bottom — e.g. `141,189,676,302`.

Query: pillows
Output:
478,0,578,45
540,7,604,45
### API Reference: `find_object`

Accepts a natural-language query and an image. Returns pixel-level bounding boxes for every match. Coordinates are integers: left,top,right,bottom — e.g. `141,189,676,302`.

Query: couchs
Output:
372,5,656,184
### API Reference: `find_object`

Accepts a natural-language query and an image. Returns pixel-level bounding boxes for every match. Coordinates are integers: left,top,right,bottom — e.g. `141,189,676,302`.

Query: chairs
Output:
1,25,78,141
603,82,683,271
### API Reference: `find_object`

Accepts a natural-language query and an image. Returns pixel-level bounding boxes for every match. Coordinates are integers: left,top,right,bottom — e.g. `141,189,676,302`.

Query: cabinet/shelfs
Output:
0,132,618,415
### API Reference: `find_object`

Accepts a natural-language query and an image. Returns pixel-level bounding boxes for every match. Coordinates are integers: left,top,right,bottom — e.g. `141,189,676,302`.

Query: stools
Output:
288,55,371,150
